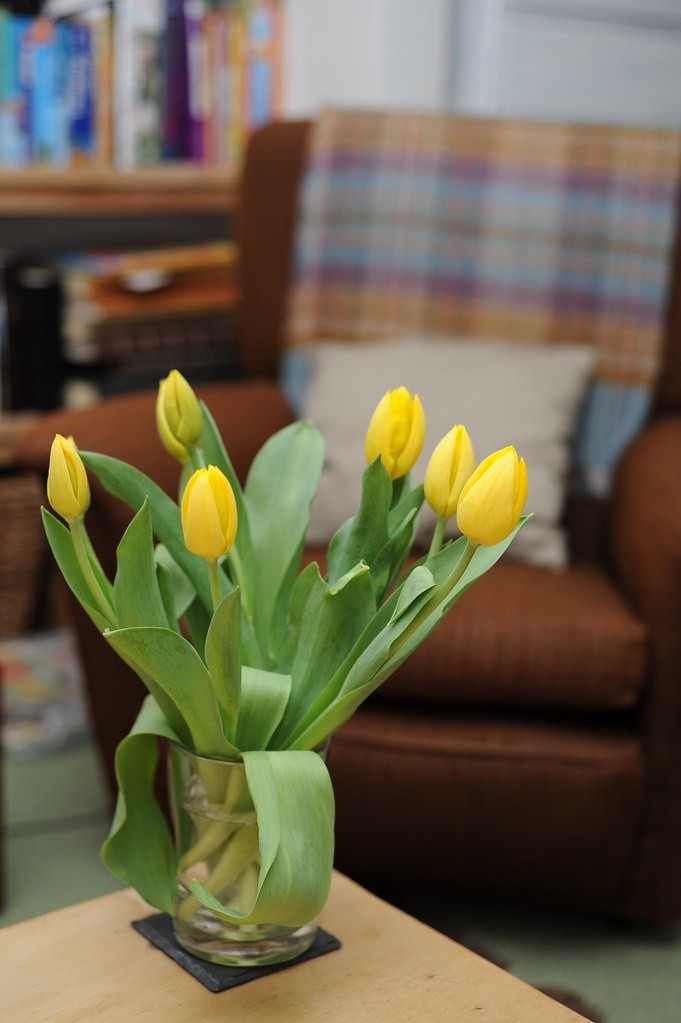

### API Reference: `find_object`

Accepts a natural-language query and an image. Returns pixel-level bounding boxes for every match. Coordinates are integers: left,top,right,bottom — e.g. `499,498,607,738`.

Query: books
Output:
58,235,245,410
0,0,286,174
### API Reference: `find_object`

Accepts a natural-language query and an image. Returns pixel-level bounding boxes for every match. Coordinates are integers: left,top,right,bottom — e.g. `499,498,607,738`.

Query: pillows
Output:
287,340,599,566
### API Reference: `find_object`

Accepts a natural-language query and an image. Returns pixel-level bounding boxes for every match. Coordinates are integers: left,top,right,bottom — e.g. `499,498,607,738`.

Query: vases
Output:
163,736,331,960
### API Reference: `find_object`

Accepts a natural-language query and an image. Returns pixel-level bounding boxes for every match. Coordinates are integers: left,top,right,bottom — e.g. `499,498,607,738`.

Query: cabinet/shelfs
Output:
0,169,238,640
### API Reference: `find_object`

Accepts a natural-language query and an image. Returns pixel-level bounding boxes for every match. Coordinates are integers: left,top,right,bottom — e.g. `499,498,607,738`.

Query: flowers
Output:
36,368,537,928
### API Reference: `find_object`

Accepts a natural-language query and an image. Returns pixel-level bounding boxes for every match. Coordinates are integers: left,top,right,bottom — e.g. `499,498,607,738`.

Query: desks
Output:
0,862,596,1023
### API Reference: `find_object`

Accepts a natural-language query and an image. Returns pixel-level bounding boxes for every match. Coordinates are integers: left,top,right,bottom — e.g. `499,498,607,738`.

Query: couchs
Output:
0,111,681,940
